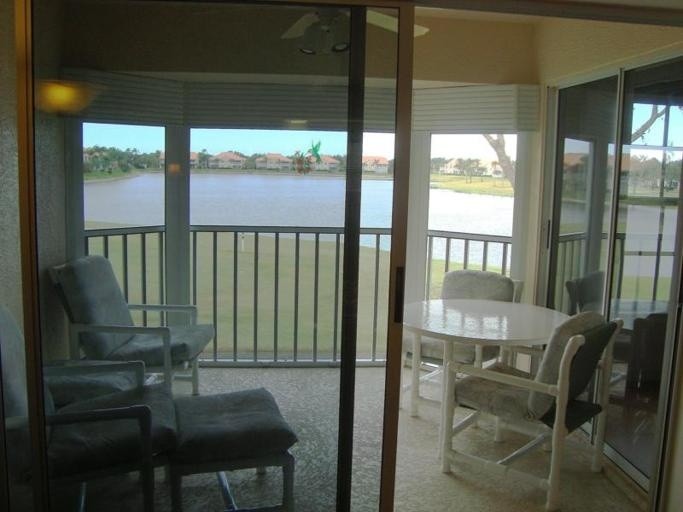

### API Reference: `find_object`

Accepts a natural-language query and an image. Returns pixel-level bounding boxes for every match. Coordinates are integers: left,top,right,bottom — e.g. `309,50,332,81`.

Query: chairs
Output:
609,314,667,404
402,271,527,445
564,270,613,315
0,301,179,509
47,255,218,396
441,309,624,512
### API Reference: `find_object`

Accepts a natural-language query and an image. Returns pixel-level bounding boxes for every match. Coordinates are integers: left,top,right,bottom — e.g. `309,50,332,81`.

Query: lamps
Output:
300,14,353,59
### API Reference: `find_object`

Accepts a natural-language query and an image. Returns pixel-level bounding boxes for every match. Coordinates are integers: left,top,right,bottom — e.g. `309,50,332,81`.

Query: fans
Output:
278,0,432,59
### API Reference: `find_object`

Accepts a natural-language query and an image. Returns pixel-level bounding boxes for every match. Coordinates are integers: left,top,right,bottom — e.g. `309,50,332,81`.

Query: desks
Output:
402,296,573,443
583,301,666,389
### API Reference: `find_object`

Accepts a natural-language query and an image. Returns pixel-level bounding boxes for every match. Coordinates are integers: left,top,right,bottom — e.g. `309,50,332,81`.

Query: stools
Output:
171,384,297,510
41,358,143,412
